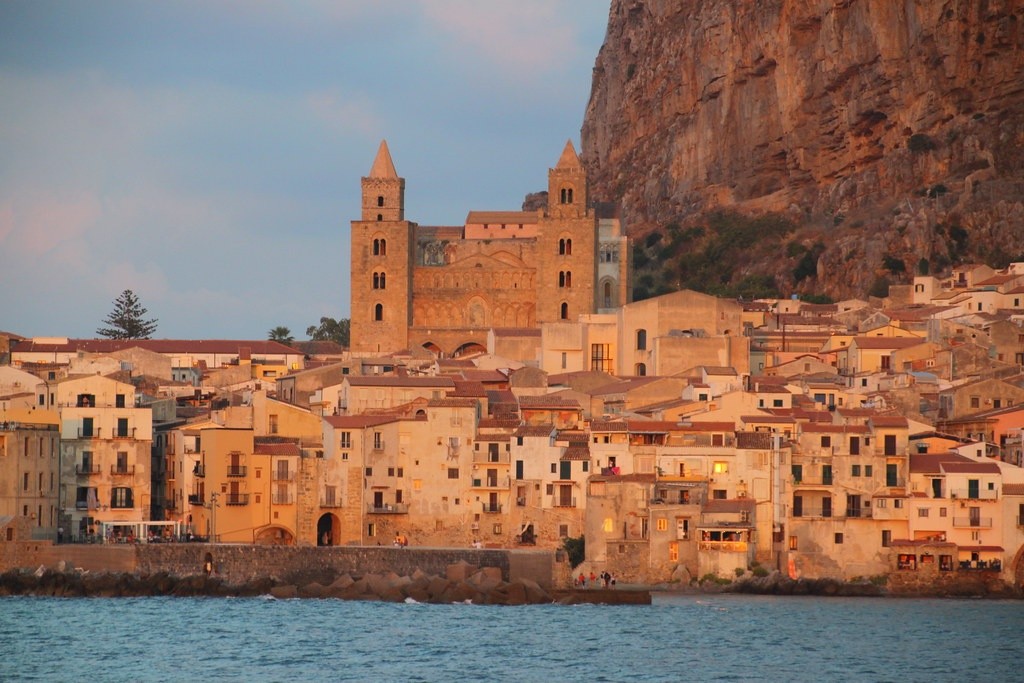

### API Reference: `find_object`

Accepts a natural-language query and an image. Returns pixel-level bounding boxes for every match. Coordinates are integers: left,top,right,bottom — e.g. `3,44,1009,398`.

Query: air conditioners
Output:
951,492,957,497
471,464,478,469
471,522,478,529
12,381,19,386
739,509,746,521
736,490,745,497
812,459,820,464
959,502,967,507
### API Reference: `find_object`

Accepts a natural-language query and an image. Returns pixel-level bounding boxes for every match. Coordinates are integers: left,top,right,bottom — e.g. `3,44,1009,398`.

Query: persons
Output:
148,528,178,543
187,529,196,541
106,528,136,544
574,571,615,591
